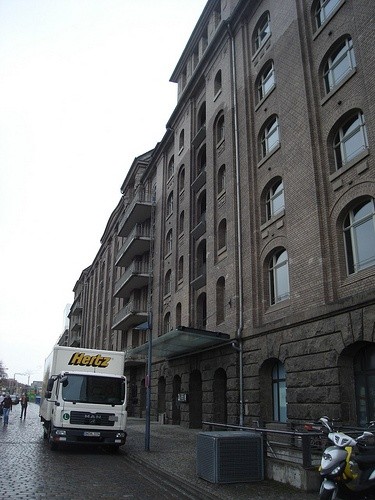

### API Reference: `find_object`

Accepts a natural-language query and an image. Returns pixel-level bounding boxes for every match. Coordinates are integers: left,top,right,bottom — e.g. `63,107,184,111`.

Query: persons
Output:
0,392,27,426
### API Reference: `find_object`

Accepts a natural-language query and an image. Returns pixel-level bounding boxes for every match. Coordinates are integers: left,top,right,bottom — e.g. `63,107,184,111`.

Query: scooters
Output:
319,416,375,500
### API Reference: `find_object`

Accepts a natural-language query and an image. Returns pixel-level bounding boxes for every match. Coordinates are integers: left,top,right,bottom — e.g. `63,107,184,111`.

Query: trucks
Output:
38,344,128,449
